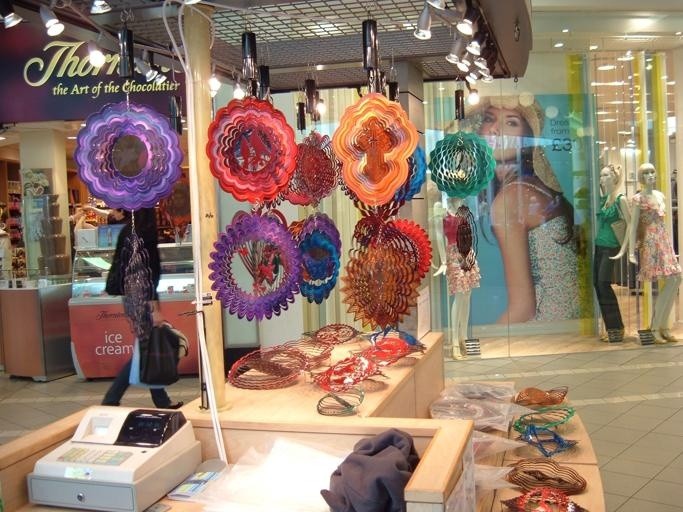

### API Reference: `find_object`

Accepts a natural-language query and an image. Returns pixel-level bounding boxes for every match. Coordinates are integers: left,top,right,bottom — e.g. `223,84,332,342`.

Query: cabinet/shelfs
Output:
0,160,89,277
363,332,448,418
66,247,200,380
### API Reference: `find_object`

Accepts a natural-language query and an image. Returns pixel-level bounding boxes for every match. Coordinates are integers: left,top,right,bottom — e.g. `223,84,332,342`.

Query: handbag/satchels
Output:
128,326,166,389
610,193,629,252
138,327,179,384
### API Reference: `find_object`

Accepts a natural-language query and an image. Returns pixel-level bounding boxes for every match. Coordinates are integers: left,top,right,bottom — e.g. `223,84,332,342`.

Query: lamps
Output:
413,1,497,122
168,42,183,136
1,1,24,31
87,32,106,67
115,1,134,79
39,0,65,37
236,7,399,136
134,49,167,85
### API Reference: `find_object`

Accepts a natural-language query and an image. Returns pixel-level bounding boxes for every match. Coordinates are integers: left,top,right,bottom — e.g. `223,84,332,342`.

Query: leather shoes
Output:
660,328,677,342
452,346,462,360
165,401,183,409
651,330,667,343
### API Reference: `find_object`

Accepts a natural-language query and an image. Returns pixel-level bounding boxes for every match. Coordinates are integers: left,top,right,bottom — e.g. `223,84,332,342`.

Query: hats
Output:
445,94,564,194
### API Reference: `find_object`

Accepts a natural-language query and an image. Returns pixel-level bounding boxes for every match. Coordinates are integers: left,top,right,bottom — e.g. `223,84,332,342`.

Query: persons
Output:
591,162,632,344
100,201,187,409
628,161,682,345
463,95,595,328
82,205,130,225
